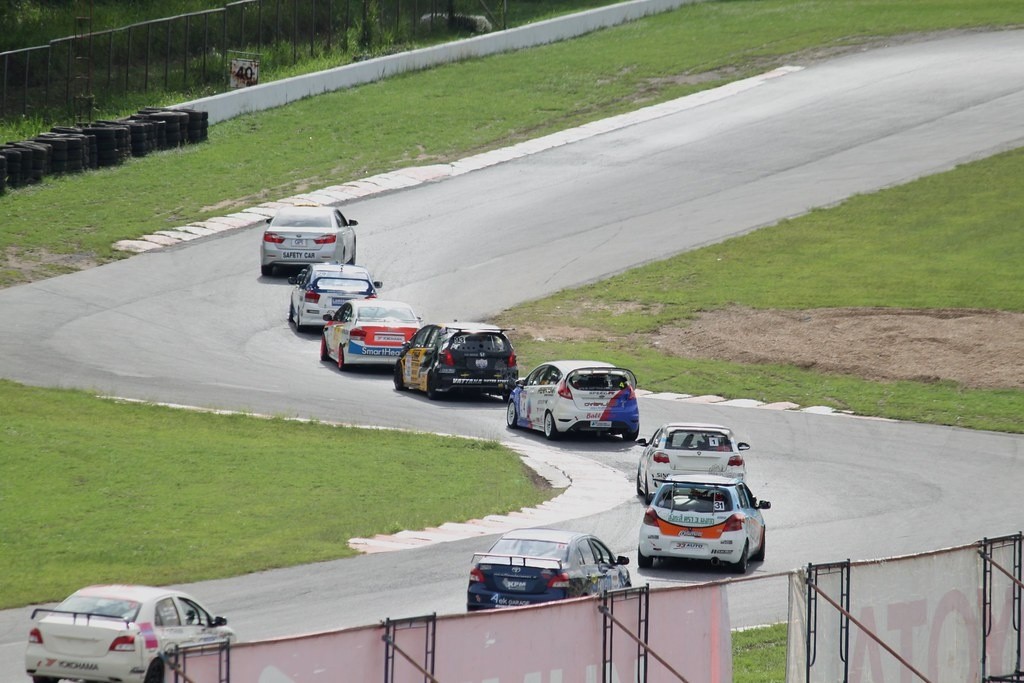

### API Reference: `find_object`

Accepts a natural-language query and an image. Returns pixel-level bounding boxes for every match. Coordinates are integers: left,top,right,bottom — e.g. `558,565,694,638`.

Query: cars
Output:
635,423,750,503
393,320,519,401
636,472,771,573
507,360,640,442
24,583,236,683
320,298,419,371
464,528,632,613
287,263,384,332
259,204,359,276
